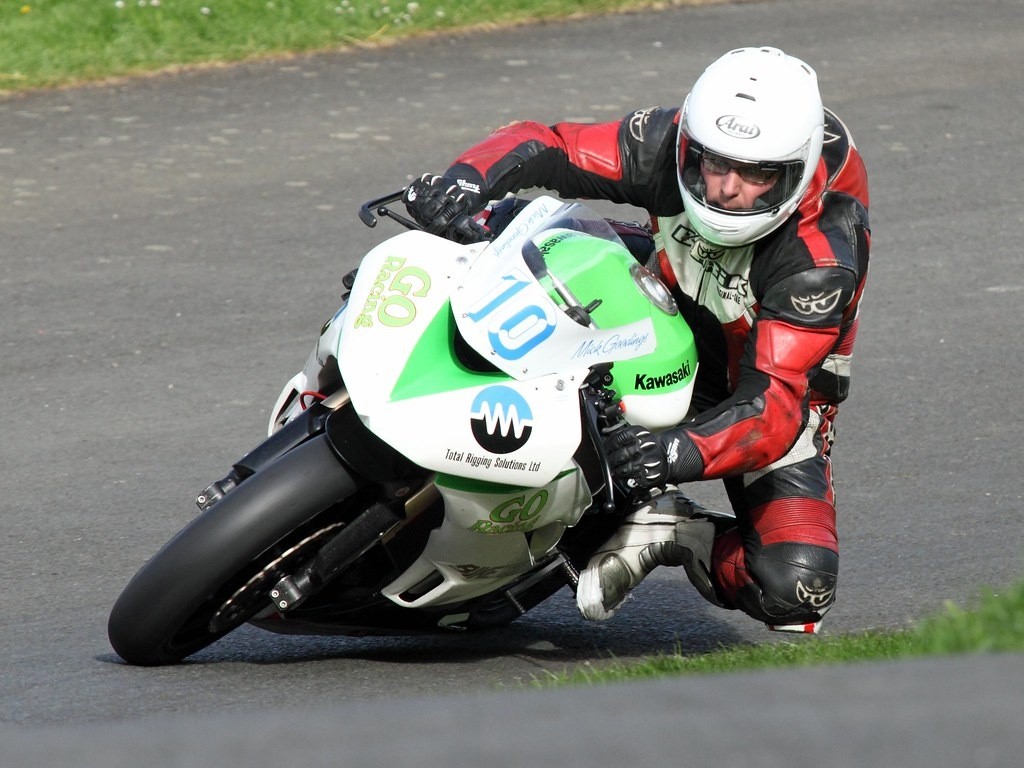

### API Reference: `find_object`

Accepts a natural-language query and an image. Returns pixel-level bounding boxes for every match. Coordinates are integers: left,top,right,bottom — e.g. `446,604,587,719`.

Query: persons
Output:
403,46,871,636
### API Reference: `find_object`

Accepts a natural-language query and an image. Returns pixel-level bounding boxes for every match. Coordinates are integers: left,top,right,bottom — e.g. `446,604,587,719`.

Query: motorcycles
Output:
107,171,699,665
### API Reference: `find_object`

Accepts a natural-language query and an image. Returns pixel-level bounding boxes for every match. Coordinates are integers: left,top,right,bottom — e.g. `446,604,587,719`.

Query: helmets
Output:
675,45,825,248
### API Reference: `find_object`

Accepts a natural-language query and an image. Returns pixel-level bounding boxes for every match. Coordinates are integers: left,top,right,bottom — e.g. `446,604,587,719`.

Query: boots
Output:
576,490,736,621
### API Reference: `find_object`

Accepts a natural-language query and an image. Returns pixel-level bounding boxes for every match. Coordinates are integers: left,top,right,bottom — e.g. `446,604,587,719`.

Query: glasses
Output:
699,157,780,184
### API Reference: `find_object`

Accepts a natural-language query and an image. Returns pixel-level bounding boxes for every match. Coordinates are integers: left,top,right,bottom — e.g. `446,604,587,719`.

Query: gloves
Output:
604,424,705,487
405,164,489,235
